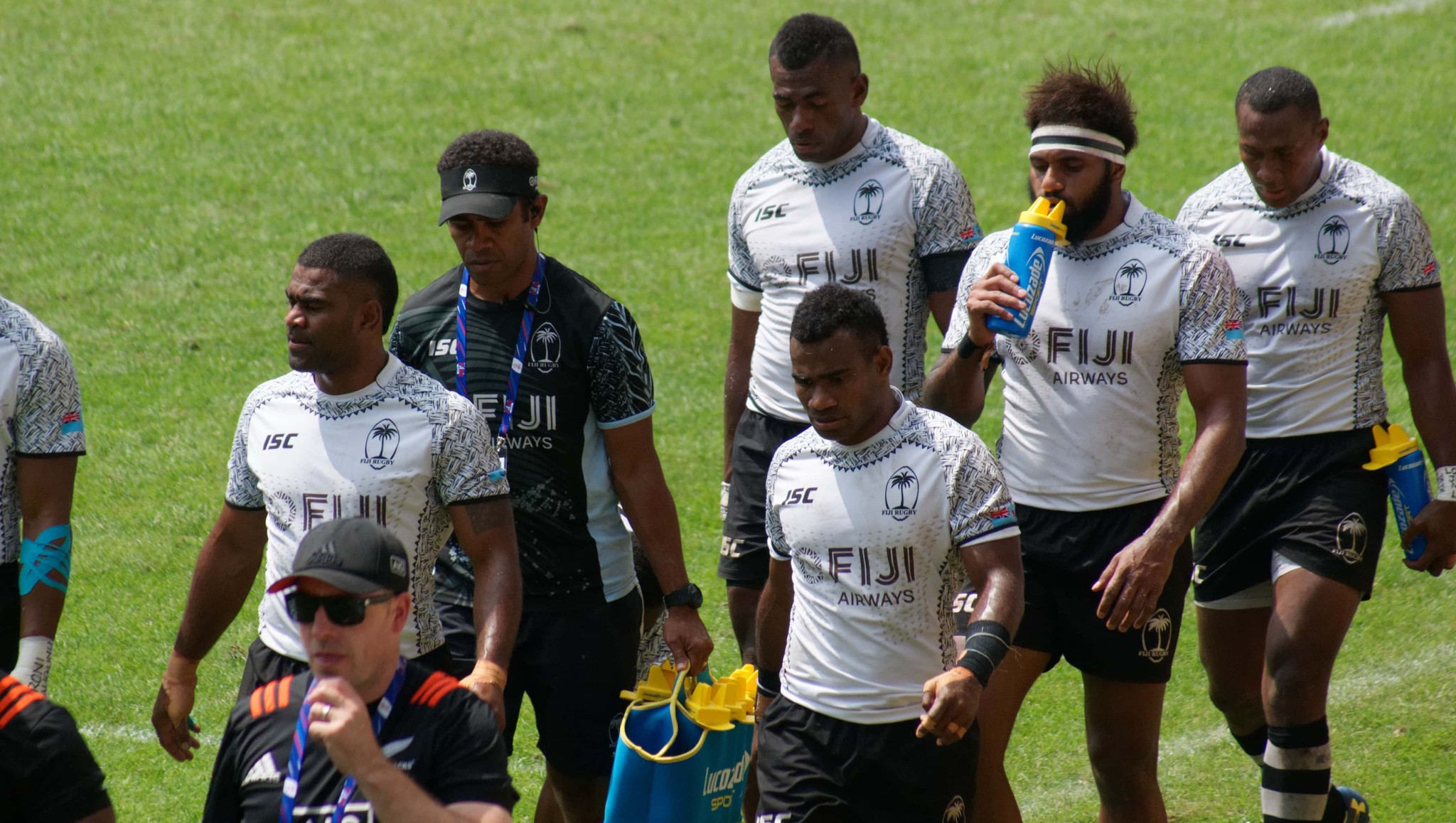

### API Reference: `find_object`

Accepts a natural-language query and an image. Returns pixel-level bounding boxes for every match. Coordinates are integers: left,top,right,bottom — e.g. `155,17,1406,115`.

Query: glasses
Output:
284,589,406,626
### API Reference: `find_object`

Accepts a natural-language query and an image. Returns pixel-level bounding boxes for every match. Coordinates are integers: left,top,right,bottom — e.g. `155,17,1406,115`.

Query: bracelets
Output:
957,329,995,362
956,620,1013,691
753,663,780,701
1435,463,1456,503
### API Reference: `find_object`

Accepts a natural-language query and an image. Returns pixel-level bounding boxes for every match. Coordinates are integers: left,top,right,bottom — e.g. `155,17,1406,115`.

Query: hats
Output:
438,165,538,226
265,516,409,594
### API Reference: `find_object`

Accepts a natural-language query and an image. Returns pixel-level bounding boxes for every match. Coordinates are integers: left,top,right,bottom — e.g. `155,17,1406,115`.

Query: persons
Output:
388,129,713,823
918,67,1251,823
717,14,1001,668
1172,66,1456,823
748,283,1026,823
0,295,117,823
204,517,522,823
150,233,524,765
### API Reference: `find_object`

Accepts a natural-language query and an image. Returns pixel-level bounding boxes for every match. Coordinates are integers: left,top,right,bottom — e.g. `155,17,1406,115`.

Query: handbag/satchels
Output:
603,659,754,823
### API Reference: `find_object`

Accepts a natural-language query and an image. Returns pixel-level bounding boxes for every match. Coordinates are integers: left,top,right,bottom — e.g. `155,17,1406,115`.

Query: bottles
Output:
985,196,1070,339
620,661,759,731
1363,423,1434,562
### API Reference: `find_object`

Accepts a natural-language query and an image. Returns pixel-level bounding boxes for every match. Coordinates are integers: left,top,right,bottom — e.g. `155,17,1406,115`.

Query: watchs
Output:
662,583,703,609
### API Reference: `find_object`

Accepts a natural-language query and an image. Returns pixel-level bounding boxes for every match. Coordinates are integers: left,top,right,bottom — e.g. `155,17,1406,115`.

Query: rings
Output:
319,704,332,722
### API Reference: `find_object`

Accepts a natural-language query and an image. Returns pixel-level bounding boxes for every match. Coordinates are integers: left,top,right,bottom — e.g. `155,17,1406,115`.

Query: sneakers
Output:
1338,786,1369,823
